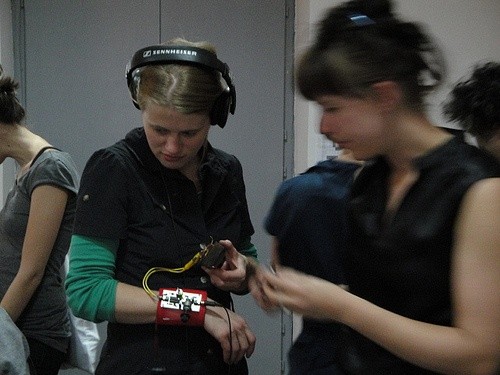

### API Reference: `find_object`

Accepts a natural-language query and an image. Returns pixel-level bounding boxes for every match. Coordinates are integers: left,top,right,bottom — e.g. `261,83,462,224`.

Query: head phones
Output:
125,45,236,128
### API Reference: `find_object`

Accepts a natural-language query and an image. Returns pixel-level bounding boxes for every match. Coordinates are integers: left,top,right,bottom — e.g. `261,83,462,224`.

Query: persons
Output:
254,1,500,375
0,64,79,375
64,38,256,375
